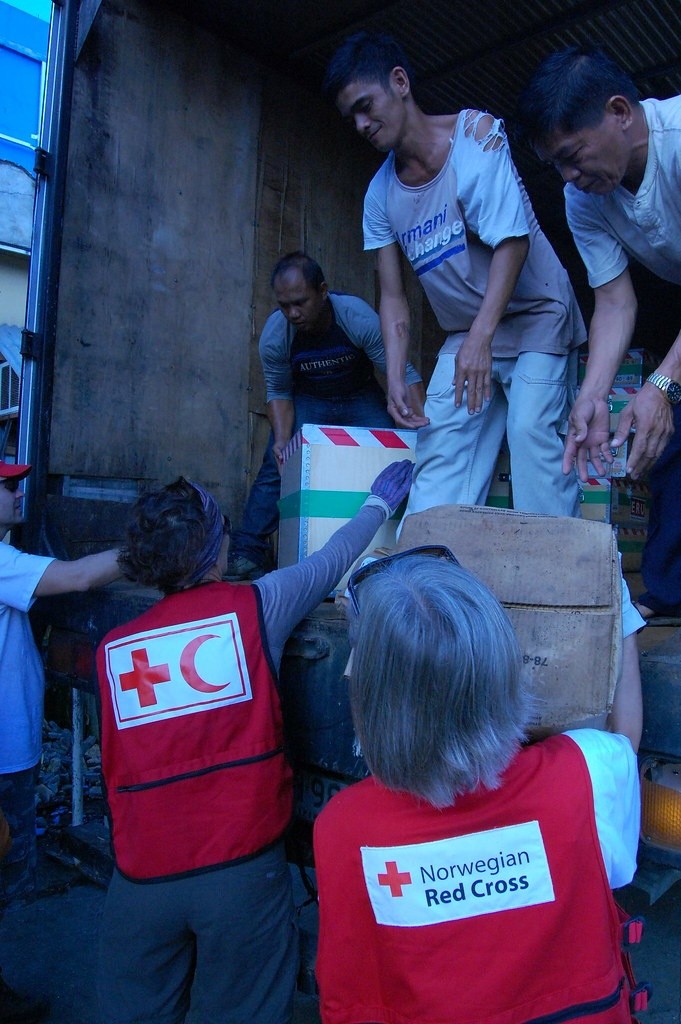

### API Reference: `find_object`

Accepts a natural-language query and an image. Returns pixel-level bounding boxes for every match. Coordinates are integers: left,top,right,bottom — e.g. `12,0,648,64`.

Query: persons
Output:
97,458,415,1024
221,253,426,581
311,554,651,1024
0,457,129,903
522,46,681,619
325,29,586,544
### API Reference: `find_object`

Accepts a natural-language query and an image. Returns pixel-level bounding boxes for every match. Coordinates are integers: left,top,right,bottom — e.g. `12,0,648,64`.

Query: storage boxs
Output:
277,348,658,727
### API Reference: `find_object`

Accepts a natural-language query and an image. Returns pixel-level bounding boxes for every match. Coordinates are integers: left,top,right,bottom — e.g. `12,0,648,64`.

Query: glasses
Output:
347,545,459,618
220,513,233,535
0,478,20,493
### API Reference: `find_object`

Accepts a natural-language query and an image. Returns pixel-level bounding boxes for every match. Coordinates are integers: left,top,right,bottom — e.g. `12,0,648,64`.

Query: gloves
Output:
362,459,415,521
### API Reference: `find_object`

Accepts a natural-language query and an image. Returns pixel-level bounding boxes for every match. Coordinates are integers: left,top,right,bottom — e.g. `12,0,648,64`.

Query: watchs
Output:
645,373,680,405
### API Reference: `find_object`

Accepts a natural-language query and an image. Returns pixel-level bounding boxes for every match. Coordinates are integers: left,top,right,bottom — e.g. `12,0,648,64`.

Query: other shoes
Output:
626,591,680,628
217,552,264,581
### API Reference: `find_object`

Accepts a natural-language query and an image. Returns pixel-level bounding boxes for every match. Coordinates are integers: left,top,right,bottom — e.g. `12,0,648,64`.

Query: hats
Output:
0,459,32,481
